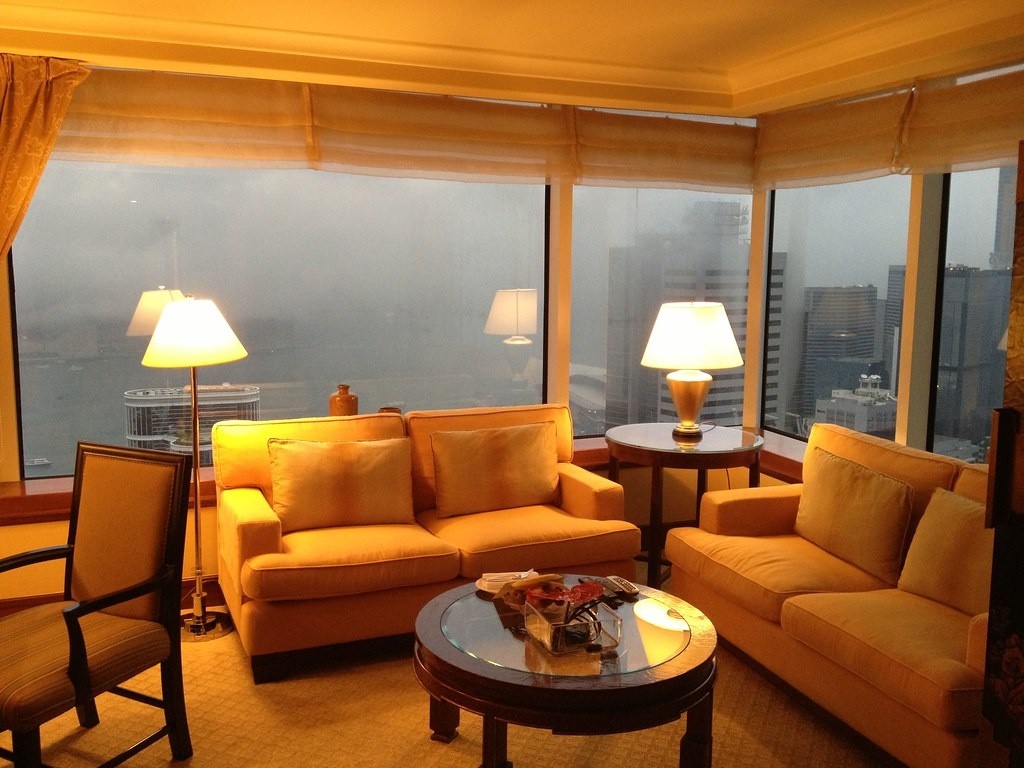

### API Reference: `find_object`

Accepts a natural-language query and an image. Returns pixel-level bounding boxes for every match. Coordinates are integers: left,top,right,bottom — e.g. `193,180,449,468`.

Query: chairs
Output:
0,440,195,767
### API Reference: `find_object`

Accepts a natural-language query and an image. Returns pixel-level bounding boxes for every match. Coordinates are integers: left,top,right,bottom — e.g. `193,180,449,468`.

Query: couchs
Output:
663,418,1023,767
207,403,646,682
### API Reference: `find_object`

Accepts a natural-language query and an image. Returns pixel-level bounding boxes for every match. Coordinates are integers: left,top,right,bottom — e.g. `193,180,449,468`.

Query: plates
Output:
476,572,538,594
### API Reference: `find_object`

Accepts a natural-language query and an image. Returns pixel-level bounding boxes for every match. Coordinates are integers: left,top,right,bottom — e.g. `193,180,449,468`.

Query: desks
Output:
600,422,765,587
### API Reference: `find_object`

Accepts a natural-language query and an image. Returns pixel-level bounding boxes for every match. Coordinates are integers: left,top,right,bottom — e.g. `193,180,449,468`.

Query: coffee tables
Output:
412,573,719,768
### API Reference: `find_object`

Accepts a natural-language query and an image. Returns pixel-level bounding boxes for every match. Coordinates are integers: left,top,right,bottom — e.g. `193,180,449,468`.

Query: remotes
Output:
578,575,640,601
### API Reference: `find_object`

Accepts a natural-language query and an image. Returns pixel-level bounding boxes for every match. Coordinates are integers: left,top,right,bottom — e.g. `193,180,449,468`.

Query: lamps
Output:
640,302,743,442
484,287,541,386
124,285,185,337
142,299,252,642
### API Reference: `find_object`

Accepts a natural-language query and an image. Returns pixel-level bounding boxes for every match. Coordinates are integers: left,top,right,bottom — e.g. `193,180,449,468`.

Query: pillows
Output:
798,450,915,588
261,434,416,535
898,490,1000,615
430,428,564,521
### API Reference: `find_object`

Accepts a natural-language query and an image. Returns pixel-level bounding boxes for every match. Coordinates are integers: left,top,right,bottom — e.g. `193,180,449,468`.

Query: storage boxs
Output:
523,600,623,654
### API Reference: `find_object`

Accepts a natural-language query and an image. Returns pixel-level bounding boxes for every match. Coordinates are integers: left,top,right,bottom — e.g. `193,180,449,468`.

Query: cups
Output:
378,407,401,413
